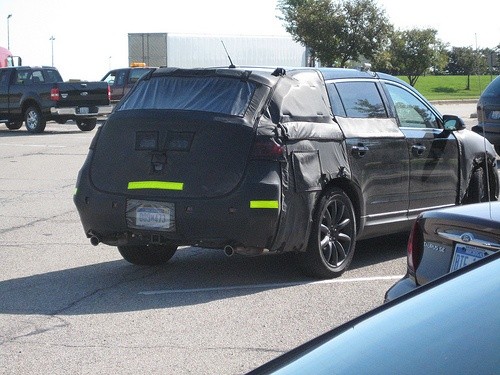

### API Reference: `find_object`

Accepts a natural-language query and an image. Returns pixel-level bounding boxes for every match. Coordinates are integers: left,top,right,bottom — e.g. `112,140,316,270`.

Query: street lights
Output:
49,36,56,67
7,14,13,50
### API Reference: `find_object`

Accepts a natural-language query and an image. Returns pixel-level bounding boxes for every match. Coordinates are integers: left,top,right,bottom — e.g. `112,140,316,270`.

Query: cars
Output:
382,197,500,303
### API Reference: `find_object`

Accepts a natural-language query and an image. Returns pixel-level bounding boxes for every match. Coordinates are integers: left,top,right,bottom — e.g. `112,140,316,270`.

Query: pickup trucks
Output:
0,66,113,133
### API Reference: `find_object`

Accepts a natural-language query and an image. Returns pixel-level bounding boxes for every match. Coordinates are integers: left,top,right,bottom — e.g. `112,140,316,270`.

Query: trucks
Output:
127,32,319,68
0,47,22,67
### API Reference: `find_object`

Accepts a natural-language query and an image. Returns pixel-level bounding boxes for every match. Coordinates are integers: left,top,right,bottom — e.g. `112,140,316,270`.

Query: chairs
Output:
31,76,42,83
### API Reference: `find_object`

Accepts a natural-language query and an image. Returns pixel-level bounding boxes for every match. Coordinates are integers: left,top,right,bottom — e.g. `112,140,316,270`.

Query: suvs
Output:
98,63,168,123
73,64,500,279
469,75,500,157
